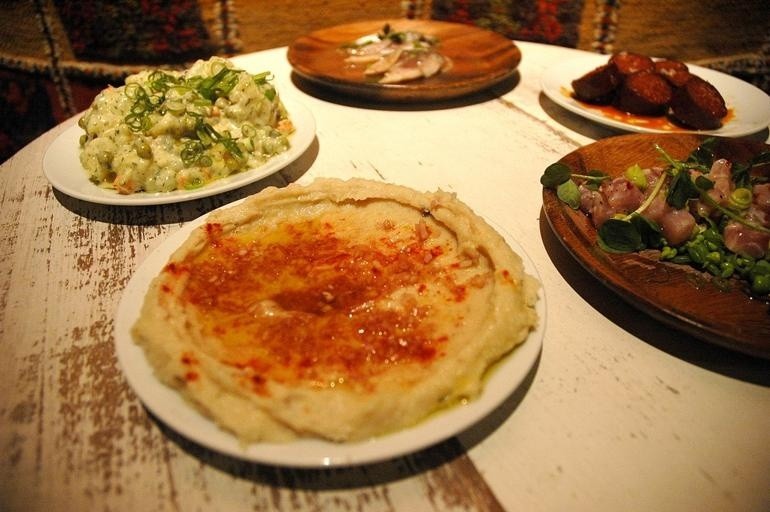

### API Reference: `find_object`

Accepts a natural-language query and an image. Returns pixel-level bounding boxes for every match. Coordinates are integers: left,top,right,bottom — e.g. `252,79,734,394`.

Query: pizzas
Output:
130,176,544,449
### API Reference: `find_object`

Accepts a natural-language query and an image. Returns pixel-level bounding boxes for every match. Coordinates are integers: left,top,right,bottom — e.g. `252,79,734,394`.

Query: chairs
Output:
1,1,242,166
683,40,769,96
403,0,621,55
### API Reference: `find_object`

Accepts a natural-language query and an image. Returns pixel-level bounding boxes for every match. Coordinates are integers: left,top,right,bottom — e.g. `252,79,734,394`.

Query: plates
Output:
112,187,547,471
538,56,770,137
42,101,317,209
286,19,523,105
541,130,770,360
